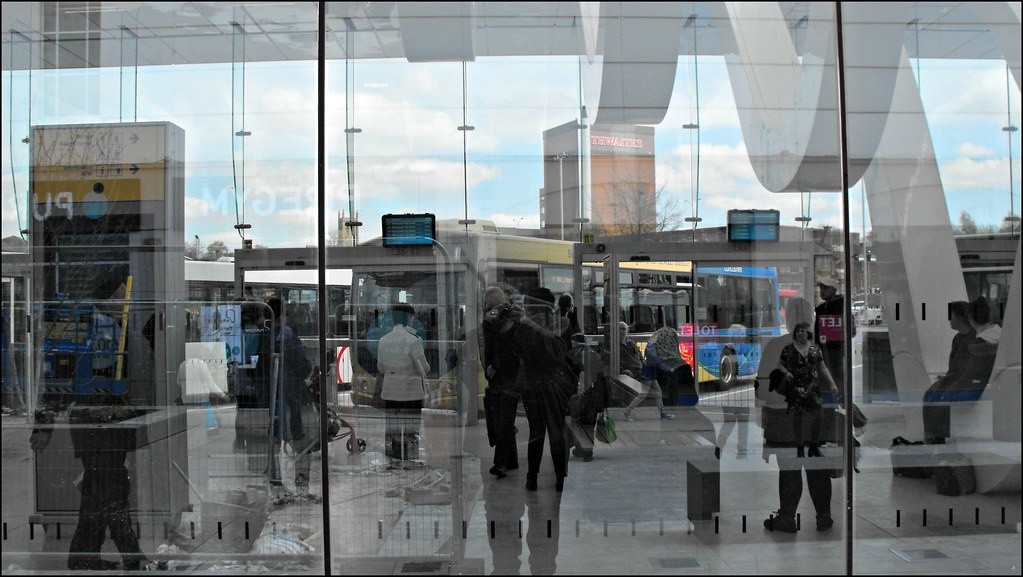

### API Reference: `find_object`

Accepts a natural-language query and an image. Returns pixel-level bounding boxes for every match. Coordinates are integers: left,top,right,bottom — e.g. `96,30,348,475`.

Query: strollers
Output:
283,345,368,458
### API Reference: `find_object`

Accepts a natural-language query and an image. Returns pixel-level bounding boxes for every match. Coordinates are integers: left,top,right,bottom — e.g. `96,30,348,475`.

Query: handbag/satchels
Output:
596,406,619,443
371,373,384,409
934,453,976,497
834,392,867,427
889,436,933,479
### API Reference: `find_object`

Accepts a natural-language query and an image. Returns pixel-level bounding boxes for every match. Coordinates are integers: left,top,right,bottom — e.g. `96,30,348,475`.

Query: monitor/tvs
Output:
727,209,780,242
382,214,436,248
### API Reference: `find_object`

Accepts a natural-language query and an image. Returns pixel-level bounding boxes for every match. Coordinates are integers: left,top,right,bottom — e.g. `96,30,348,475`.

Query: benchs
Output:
562,373,715,461
686,431,1021,544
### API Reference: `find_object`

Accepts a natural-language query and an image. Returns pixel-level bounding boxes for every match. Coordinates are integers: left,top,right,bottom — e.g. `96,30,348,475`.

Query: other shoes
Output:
621,412,634,421
404,457,431,469
762,514,797,533
123,559,168,571
815,514,834,532
69,554,121,571
660,412,676,419
571,448,593,462
525,472,538,491
554,471,565,492
385,457,405,469
490,460,519,473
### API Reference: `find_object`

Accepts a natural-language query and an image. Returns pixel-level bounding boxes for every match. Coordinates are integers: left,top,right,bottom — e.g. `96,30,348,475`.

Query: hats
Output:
816,278,838,290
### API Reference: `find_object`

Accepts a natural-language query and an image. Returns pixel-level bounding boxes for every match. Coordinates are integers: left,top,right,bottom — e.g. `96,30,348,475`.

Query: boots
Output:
269,480,295,504
294,474,320,504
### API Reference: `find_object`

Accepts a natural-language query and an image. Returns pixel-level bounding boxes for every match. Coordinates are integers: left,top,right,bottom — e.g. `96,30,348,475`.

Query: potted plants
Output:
1,406,190,539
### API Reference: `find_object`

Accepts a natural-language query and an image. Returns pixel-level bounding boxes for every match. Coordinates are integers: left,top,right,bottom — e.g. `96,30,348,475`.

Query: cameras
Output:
500,303,511,317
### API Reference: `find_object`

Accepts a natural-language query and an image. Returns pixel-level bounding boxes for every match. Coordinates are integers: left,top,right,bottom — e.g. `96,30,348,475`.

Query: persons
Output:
809,277,858,389
617,321,647,378
227,292,322,507
27,278,169,569
779,320,838,455
752,294,846,532
712,325,758,459
378,303,431,469
439,283,604,577
624,332,677,419
449,281,588,493
917,297,1002,449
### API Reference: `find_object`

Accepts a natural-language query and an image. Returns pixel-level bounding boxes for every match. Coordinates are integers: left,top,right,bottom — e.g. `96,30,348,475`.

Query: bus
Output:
185,257,354,403
336,220,798,420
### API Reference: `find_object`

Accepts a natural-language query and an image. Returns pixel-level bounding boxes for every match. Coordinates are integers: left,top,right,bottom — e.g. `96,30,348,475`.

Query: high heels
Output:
808,448,825,458
796,447,806,458
